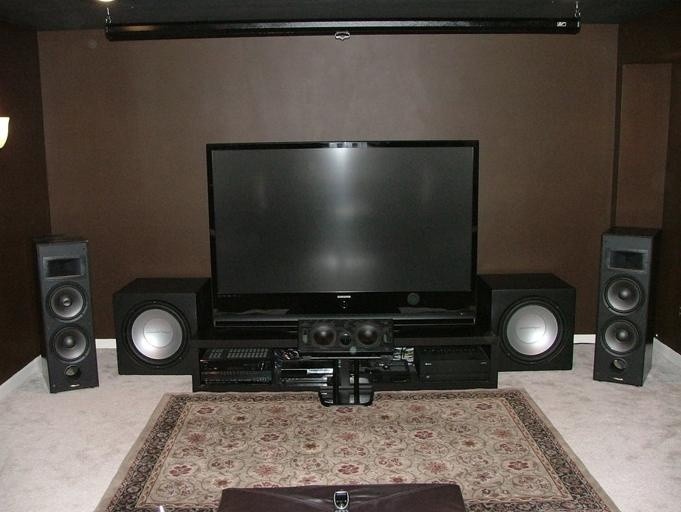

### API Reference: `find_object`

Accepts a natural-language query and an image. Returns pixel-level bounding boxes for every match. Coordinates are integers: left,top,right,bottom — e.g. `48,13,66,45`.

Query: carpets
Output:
93,385,620,511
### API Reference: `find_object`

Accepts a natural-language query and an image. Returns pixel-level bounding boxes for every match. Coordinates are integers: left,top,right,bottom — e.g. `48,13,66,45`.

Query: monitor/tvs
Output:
204,140,479,330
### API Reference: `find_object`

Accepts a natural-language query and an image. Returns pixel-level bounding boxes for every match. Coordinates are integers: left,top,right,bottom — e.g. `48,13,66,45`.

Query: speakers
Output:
592,225,663,387
478,271,577,372
296,318,395,355
29,238,99,393
112,278,211,375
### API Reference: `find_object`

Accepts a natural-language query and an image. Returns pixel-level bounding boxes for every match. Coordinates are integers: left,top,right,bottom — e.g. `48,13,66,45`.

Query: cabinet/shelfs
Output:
187,329,499,392
